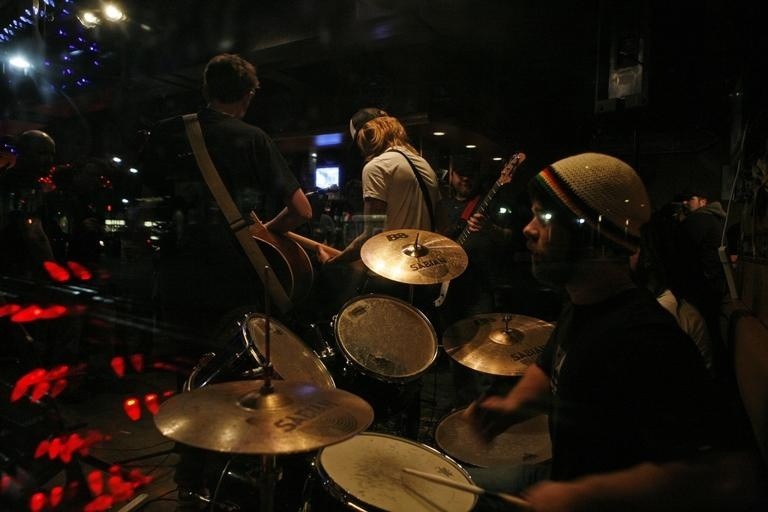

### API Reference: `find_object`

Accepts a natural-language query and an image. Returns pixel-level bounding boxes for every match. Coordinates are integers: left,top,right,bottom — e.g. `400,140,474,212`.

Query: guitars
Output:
430,152,526,307
246,222,314,319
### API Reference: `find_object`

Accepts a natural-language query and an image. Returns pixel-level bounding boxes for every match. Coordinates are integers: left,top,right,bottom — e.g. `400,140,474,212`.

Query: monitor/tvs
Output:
314,166,340,190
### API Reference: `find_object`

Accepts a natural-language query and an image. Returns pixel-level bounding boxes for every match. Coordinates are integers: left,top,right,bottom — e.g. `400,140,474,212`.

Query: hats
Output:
536,152,652,254
347,108,387,151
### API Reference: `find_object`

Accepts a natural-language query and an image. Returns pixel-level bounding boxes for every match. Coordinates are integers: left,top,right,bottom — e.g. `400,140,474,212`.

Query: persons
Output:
315,107,441,301
149,56,312,357
0,122,733,384
460,154,732,512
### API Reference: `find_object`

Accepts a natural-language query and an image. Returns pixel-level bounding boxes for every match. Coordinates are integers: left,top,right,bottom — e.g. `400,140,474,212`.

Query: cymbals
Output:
443,312,555,377
153,380,375,455
360,228,468,285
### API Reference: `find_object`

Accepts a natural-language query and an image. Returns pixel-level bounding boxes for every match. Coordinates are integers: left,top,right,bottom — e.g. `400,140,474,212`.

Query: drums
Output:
432,404,552,512
325,293,438,422
296,431,479,512
182,312,336,389
